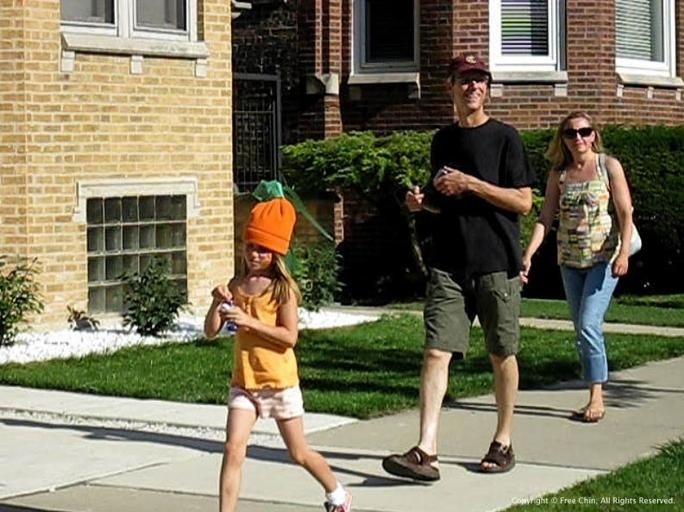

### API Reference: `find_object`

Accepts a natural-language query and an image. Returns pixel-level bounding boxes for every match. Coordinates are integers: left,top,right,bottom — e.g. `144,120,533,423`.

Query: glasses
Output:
560,127,595,139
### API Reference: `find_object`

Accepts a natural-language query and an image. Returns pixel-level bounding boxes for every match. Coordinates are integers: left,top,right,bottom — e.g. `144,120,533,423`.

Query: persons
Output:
380,54,533,483
202,196,352,512
521,109,635,423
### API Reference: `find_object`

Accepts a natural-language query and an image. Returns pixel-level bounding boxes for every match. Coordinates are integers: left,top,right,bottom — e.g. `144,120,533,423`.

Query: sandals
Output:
383,444,440,481
480,436,516,472
581,400,607,422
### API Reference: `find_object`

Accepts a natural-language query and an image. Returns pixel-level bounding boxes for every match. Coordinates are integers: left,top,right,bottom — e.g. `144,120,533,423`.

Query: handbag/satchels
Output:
628,223,642,258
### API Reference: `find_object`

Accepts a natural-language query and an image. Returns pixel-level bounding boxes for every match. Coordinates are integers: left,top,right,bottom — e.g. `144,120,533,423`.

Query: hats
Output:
447,54,490,77
245,198,296,256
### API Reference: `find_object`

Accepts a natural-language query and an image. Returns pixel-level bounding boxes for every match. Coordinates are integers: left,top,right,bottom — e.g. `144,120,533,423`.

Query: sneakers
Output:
325,489,353,512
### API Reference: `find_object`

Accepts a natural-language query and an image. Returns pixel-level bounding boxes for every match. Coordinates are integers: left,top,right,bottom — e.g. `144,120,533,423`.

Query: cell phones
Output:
432,169,450,181
